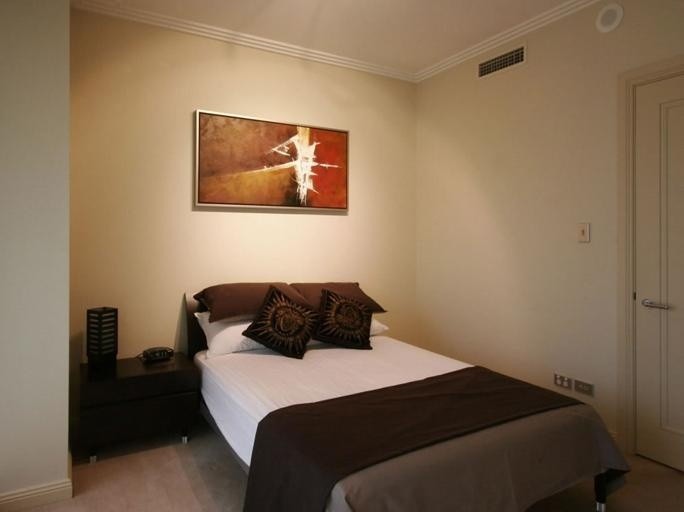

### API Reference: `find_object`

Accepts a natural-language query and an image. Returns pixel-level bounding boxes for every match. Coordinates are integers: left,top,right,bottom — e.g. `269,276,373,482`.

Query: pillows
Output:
312,288,375,350
241,284,321,360
193,281,292,324
289,282,388,314
194,309,390,360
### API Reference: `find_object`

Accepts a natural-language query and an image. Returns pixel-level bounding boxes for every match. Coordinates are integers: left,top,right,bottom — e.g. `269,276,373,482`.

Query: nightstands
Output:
79,350,202,464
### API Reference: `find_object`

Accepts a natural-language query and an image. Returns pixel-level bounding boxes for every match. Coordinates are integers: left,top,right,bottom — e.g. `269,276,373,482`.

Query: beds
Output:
185,292,631,512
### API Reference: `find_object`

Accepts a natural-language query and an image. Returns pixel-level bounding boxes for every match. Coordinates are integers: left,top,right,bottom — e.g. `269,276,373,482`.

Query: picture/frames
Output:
194,109,350,213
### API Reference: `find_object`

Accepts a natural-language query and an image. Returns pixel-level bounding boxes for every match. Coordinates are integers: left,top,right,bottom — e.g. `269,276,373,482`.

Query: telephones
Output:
137,347,173,364
